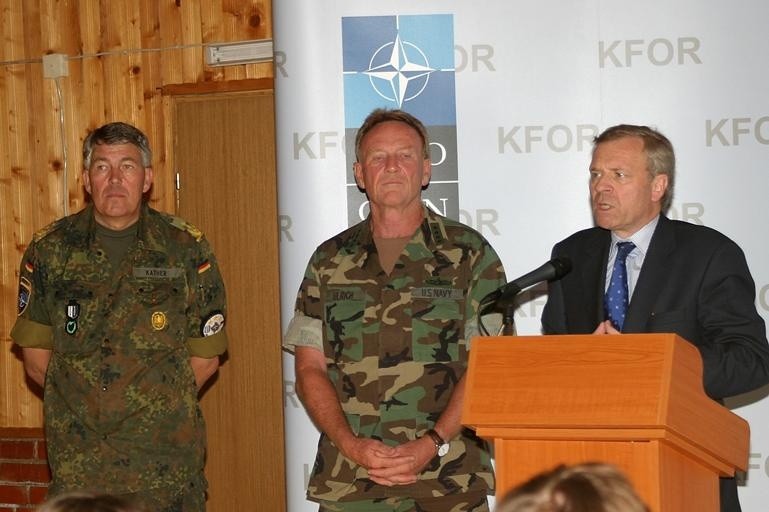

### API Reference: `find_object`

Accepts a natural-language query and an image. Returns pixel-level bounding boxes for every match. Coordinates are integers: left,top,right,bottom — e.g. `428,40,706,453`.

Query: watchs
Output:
425,427,451,459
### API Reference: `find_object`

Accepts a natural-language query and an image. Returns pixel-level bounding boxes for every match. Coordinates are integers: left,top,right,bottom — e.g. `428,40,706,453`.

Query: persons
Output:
10,122,230,511
495,459,651,512
284,108,515,512
542,125,769,512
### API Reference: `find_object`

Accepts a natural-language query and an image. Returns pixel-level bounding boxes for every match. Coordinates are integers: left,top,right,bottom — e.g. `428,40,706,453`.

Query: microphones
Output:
497,256,574,296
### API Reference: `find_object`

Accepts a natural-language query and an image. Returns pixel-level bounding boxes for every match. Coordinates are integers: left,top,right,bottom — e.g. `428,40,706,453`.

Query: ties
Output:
604,243,636,333
